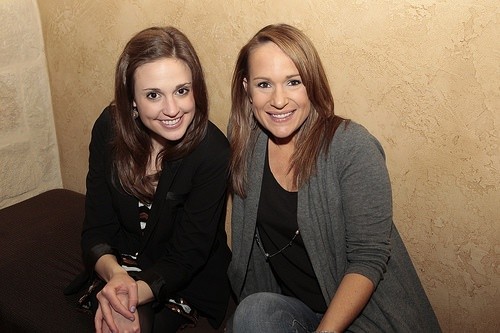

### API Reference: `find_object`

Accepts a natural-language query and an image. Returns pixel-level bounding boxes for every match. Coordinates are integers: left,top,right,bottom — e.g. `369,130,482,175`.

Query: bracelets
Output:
313,330,335,333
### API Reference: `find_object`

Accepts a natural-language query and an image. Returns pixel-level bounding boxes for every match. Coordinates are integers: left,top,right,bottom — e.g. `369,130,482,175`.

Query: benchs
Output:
0,189,240,332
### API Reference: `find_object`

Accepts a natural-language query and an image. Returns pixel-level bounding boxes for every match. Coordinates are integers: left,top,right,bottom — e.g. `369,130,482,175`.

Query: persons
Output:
63,27,232,332
225,23,442,332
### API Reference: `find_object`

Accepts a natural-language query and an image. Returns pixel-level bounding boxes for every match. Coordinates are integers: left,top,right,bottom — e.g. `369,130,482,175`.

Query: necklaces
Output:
255,228,299,258
